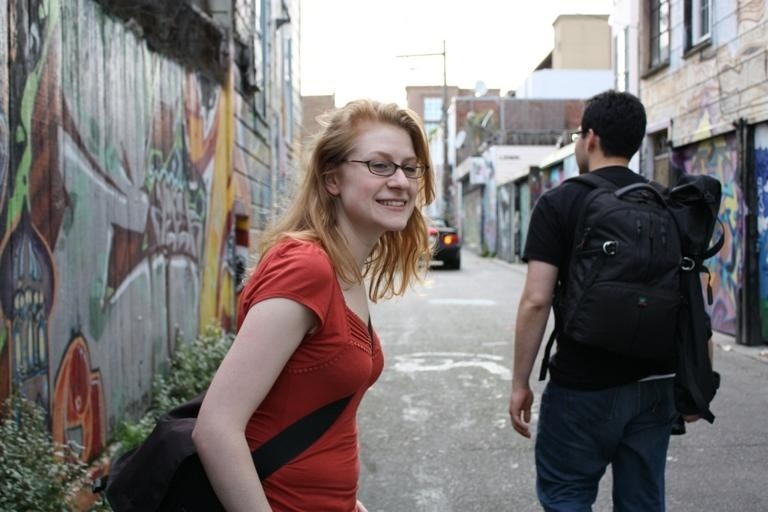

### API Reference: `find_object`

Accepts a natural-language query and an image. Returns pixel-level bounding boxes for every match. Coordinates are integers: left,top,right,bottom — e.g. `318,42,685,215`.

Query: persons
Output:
191,100,437,512
509,90,722,512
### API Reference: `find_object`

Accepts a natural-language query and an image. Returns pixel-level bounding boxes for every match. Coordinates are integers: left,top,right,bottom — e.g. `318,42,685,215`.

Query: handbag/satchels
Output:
106,389,227,512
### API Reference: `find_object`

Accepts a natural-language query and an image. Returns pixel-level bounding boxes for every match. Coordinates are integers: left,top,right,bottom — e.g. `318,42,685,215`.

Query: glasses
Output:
571,131,587,142
343,157,426,178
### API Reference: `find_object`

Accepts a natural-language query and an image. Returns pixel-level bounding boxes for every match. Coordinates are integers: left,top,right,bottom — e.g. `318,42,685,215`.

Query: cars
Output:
425,216,463,271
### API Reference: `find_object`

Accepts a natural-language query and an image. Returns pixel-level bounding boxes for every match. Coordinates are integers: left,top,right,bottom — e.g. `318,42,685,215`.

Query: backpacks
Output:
562,173,724,390
